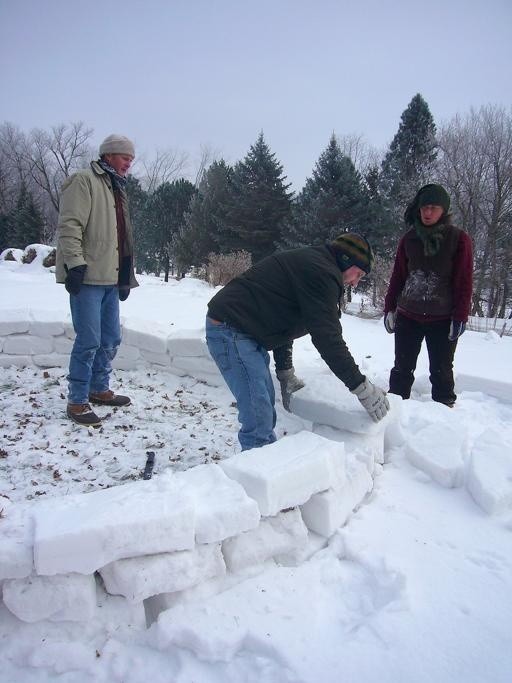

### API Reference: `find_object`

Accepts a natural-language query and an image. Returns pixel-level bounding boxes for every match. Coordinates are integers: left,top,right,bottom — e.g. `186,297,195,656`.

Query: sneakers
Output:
89,389,131,406
67,403,101,426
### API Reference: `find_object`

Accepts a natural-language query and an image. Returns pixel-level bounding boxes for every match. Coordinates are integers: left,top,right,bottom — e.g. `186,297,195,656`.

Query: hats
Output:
99,134,136,159
419,189,447,209
332,232,374,275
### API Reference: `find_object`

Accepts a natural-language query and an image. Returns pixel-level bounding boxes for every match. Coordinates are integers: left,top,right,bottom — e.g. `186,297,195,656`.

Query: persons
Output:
56,132,141,429
384,185,473,407
205,229,389,453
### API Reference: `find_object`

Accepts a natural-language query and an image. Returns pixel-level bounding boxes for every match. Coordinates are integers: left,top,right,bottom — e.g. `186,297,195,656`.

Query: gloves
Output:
383,310,396,334
275,367,305,413
447,321,466,341
349,376,390,424
119,269,131,302
64,264,87,296
